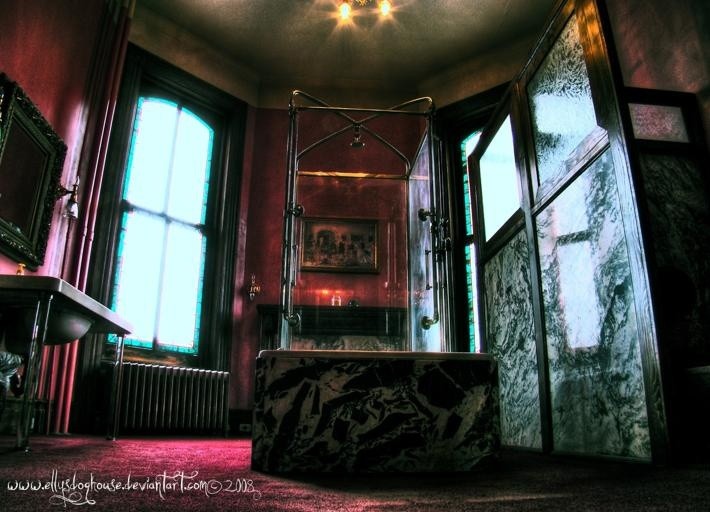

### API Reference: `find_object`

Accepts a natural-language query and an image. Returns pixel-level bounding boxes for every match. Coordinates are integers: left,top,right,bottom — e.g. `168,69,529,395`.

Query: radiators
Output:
116,361,231,437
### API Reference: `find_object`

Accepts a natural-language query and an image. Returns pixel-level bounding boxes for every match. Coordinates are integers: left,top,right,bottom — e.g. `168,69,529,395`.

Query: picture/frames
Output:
297,217,381,274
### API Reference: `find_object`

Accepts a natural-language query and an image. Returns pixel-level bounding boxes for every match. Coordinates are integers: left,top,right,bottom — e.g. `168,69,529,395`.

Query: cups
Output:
332,295,342,306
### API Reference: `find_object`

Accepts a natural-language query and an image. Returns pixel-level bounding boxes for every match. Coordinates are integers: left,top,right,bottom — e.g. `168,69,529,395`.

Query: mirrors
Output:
0,72,69,272
